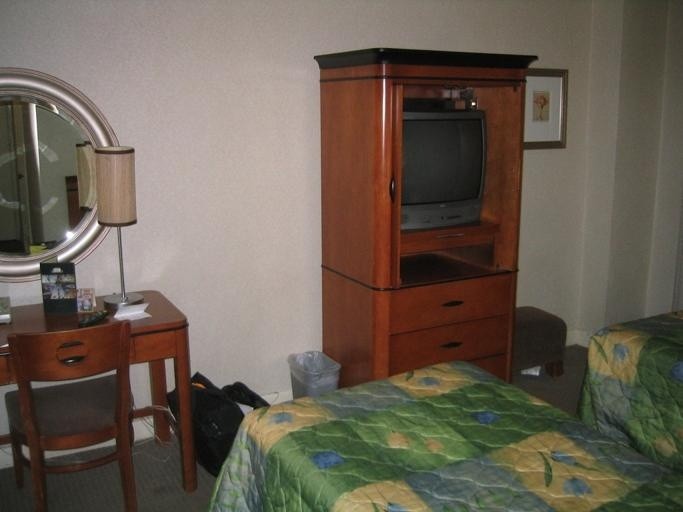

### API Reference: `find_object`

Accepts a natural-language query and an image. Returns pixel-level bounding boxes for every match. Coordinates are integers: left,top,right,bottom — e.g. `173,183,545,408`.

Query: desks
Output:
0,289,199,492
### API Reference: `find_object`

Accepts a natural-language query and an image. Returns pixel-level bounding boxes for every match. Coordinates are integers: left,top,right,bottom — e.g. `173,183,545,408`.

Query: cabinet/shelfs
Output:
316,50,539,291
316,267,519,388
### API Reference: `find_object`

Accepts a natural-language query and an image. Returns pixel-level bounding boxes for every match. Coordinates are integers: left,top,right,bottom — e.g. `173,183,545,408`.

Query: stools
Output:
511,305,569,382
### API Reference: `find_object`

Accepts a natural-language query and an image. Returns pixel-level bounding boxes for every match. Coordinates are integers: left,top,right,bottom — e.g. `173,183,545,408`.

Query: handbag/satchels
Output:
166,369,271,481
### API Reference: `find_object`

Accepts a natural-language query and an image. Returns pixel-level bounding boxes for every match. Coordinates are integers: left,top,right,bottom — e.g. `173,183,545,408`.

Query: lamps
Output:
94,144,139,307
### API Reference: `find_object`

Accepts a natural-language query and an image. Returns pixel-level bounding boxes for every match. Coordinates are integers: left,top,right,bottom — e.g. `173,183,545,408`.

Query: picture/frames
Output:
523,67,570,149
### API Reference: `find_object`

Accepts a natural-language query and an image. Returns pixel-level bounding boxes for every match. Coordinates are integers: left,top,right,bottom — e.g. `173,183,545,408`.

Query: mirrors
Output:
0,65,119,284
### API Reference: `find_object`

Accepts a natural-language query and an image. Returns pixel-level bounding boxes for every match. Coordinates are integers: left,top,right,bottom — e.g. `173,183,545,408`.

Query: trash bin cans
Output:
287,351,341,398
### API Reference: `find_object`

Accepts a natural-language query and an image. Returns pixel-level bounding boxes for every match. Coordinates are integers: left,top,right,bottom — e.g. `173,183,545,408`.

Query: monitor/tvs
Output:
399,111,488,233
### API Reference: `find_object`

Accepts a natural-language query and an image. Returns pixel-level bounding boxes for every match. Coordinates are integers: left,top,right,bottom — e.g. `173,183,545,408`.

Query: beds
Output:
211,357,681,511
578,311,682,474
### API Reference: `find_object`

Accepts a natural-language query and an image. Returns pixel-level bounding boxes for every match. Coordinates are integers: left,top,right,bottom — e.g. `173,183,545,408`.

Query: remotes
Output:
79,309,108,326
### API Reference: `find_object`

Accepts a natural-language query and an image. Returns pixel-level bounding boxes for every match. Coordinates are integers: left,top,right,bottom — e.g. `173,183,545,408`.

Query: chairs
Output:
6,319,138,511
